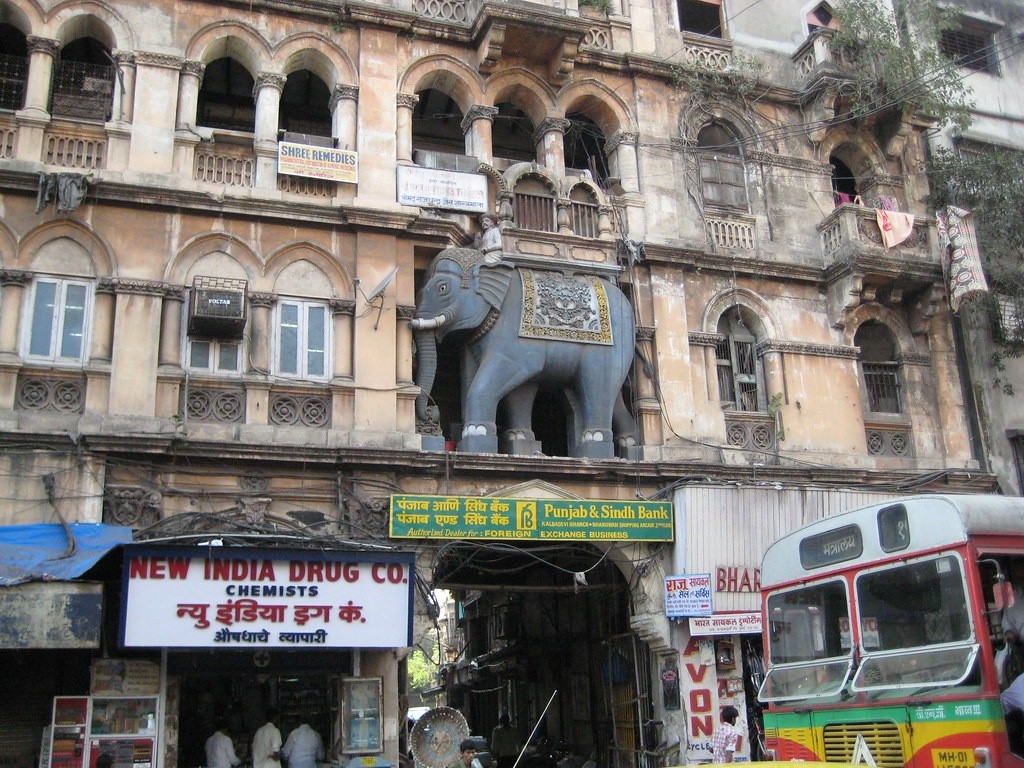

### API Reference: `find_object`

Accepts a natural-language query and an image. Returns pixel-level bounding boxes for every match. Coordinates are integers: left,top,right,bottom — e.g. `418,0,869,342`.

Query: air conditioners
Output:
187,288,248,337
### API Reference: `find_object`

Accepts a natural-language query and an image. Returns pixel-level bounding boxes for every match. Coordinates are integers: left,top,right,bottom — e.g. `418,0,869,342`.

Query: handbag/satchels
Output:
993,641,1020,691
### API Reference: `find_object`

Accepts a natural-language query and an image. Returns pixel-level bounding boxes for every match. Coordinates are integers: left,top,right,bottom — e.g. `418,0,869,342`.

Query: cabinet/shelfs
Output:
49,695,159,768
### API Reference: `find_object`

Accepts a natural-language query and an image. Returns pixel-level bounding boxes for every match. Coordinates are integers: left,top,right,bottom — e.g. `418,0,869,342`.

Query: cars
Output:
407,707,432,732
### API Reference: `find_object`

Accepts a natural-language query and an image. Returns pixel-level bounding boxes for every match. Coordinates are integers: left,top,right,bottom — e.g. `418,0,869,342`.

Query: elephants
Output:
407,247,640,446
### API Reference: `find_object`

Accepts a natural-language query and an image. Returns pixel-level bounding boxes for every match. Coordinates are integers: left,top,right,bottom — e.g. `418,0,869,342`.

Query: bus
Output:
758,494,1024,768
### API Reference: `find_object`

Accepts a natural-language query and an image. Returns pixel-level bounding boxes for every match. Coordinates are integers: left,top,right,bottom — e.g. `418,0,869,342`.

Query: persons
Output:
448,739,483,768
252,709,282,768
709,707,739,764
1000,582,1024,716
206,720,241,768
281,712,325,768
894,612,918,649
492,714,521,768
